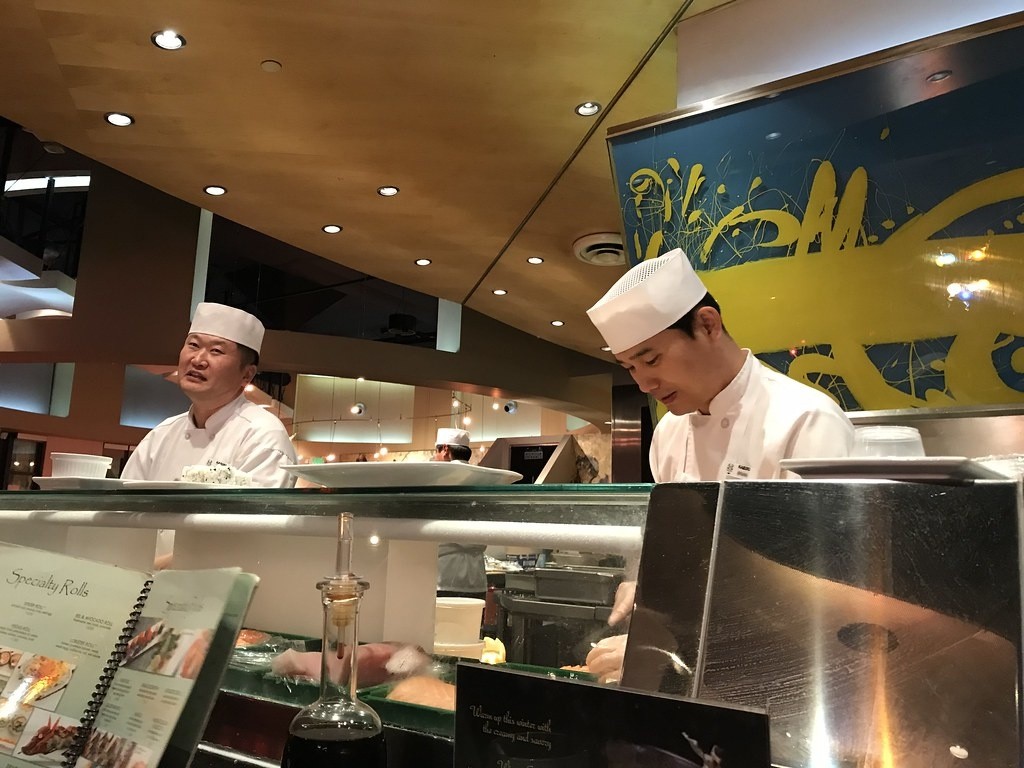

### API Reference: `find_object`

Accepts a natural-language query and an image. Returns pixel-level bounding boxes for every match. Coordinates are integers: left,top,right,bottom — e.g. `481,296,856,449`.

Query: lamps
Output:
4,168,91,196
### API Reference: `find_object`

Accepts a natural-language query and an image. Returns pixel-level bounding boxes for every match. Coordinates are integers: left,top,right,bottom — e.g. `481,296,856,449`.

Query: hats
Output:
187,302,265,356
586,248,709,354
435,427,470,447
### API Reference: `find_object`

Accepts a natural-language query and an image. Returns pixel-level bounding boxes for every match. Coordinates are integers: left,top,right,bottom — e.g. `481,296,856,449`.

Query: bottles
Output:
280,512,386,768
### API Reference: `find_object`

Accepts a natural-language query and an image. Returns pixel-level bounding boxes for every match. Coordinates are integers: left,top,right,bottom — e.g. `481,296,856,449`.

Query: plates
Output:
122,481,245,489
779,456,1011,482
234,629,272,648
120,619,166,666
278,461,522,487
33,477,134,491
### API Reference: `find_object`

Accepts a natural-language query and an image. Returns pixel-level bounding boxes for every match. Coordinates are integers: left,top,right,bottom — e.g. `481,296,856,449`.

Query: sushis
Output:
179,460,252,486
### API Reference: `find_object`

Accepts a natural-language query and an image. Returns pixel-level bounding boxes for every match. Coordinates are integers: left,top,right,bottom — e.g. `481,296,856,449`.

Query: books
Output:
0,541,261,768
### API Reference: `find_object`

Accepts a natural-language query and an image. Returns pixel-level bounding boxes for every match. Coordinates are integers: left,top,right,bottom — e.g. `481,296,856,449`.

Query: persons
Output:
586,248,857,690
428,428,488,634
120,302,300,489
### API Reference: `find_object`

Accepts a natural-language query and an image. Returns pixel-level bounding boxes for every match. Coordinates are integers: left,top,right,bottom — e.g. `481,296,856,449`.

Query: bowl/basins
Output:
435,597,486,644
435,640,485,662
855,427,926,459
50,452,112,477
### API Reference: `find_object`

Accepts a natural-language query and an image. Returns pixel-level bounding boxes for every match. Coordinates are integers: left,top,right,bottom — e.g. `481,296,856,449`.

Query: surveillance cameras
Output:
504,401,516,412
